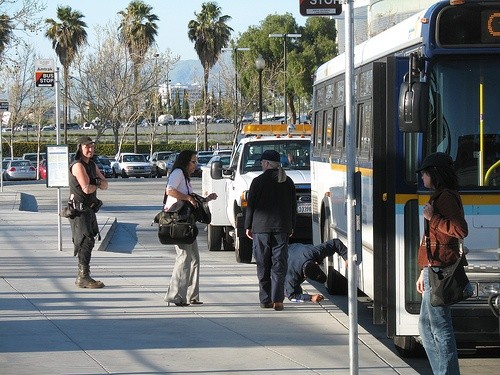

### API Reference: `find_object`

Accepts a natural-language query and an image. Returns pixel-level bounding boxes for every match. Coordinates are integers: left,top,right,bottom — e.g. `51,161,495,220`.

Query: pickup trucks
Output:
203,123,313,263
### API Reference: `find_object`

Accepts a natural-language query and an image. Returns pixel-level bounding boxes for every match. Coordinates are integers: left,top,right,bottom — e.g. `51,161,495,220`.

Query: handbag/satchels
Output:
427,253,473,307
151,208,198,244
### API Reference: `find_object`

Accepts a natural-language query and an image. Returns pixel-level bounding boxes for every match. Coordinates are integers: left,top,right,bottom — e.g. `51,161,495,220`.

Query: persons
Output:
163,150,218,308
69,137,108,289
244,150,298,309
286,238,348,302
415,152,469,375
284,153,301,167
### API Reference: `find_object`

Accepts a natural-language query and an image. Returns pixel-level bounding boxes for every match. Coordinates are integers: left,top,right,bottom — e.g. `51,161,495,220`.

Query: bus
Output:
309,0,500,358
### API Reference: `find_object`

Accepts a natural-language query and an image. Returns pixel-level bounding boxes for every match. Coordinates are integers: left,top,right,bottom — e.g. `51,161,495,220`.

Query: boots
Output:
75,263,104,289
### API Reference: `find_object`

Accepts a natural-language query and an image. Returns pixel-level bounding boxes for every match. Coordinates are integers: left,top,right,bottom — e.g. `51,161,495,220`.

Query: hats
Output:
77,136,95,145
304,263,327,283
258,150,281,162
416,152,452,173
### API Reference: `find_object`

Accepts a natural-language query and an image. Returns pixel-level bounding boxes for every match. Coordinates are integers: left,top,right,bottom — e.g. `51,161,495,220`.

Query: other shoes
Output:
261,302,272,308
273,301,284,311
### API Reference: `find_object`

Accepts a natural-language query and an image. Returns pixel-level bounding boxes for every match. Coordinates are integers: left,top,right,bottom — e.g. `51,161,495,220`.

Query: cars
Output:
41,123,80,131
110,153,153,178
148,151,172,178
13,124,34,132
3,160,36,181
195,149,231,178
165,154,201,178
176,119,190,125
2,153,46,167
39,158,46,179
2,128,11,132
69,153,115,178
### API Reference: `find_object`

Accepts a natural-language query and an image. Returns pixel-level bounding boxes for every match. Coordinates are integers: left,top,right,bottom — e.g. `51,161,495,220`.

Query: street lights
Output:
220,47,253,129
153,53,159,124
255,58,266,125
268,33,301,124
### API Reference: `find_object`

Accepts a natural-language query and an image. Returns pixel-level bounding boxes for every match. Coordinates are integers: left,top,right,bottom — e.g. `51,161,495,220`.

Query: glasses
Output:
189,160,196,164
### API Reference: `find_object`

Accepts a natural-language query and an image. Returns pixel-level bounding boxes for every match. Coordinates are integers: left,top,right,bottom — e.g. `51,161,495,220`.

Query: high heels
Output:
167,300,189,306
189,299,203,304
